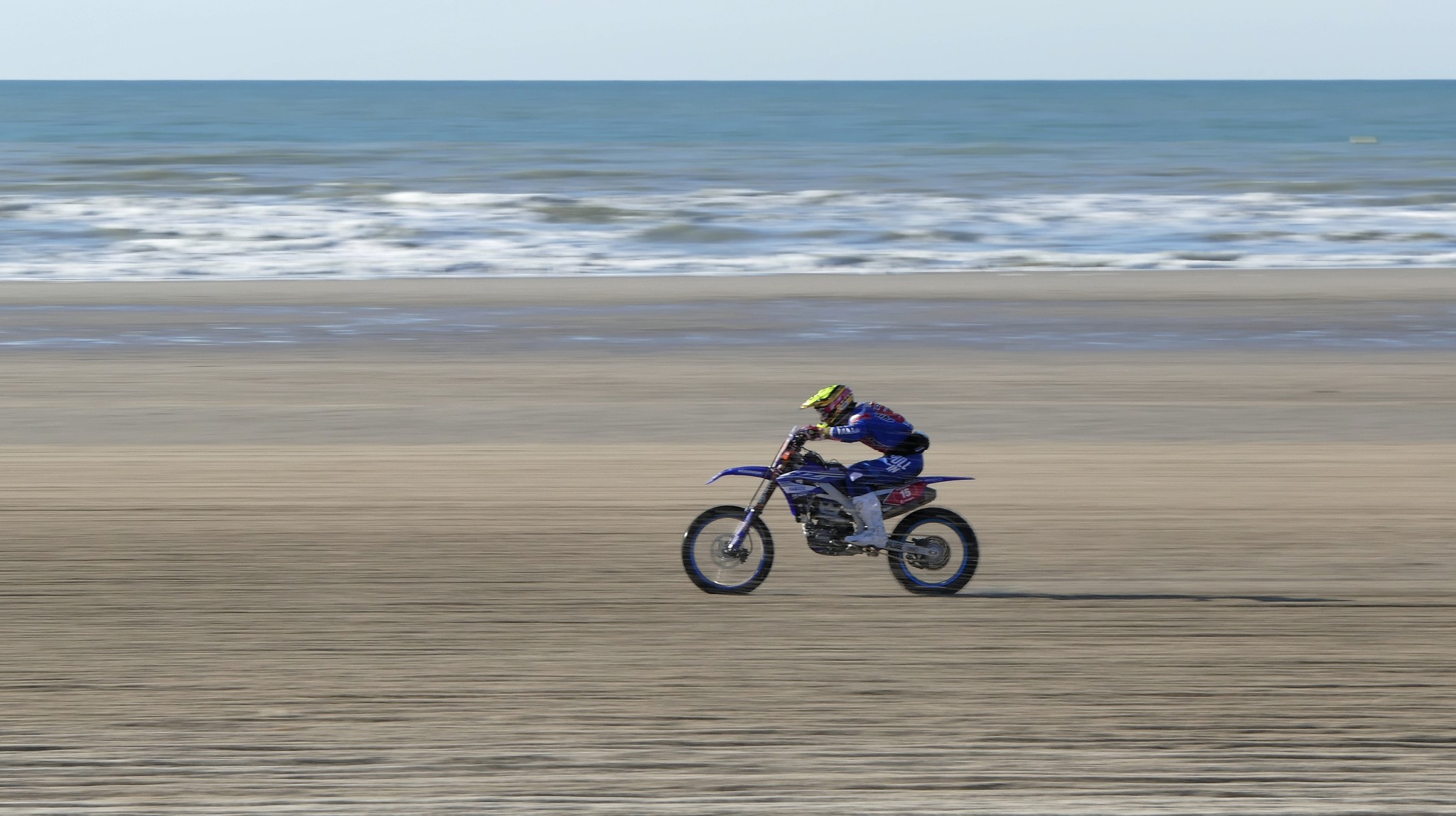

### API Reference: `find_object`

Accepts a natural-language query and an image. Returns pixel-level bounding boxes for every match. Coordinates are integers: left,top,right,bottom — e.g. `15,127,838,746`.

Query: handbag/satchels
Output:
892,430,929,455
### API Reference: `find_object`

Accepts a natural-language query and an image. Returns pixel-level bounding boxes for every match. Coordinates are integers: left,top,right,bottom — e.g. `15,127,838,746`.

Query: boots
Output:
845,492,888,546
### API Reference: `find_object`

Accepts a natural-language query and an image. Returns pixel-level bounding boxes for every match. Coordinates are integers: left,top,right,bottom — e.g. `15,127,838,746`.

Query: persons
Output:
795,383,925,549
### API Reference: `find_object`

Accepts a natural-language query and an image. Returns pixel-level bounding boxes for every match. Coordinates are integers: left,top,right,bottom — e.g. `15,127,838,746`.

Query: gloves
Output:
795,425,823,439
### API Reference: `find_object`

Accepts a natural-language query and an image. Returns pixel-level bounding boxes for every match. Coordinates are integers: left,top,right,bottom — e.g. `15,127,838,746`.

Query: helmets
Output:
800,383,854,428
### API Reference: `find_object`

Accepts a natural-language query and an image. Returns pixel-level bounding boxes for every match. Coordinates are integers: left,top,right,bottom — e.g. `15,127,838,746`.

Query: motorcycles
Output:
679,424,980,598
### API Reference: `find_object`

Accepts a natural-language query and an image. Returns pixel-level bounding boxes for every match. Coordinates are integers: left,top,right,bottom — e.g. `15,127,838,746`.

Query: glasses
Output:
818,409,828,421
